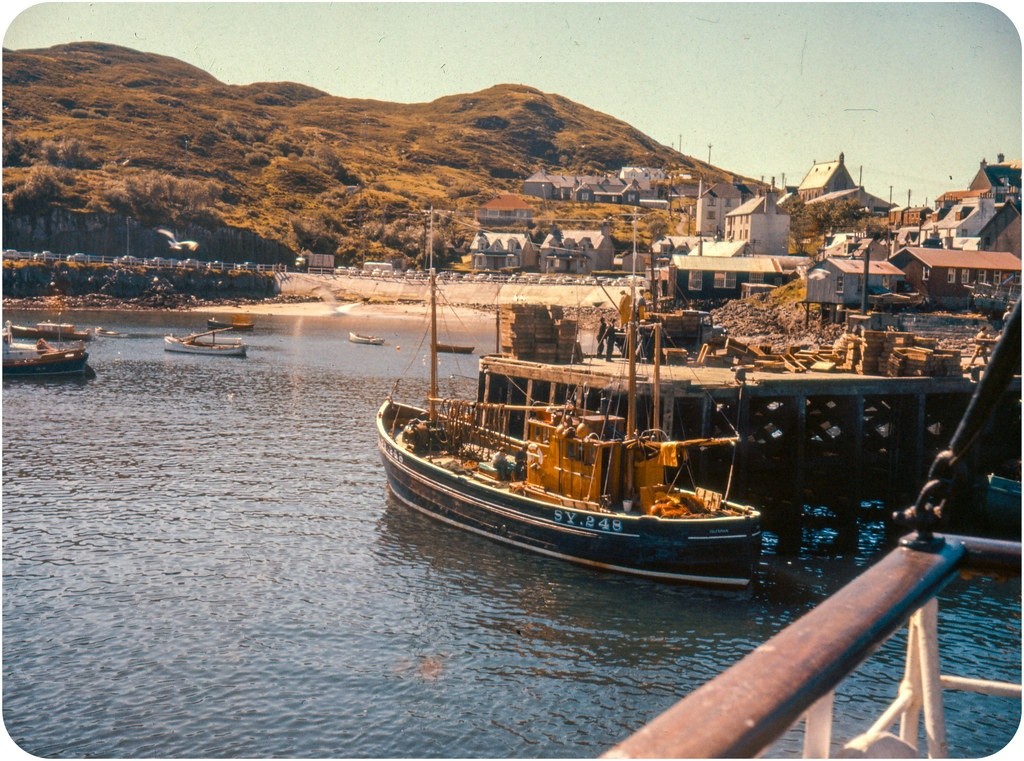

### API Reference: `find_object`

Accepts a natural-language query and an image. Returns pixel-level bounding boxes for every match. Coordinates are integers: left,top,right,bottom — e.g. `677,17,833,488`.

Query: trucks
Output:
613,308,728,358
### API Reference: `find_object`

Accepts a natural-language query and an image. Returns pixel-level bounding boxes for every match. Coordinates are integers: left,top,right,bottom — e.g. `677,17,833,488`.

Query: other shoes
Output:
597,354,603,359
606,359,614,362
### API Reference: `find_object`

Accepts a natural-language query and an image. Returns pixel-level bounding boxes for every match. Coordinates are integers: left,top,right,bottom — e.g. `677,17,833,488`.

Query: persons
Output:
596,316,607,359
492,446,511,479
604,318,615,362
515,446,527,479
970,304,1013,365
637,288,647,320
618,290,632,331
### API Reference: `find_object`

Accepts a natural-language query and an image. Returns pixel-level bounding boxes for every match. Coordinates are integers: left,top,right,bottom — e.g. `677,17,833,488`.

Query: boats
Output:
375,205,762,589
348,330,387,346
2,327,88,376
94,326,129,338
10,319,92,340
206,315,255,334
162,333,251,356
429,340,477,356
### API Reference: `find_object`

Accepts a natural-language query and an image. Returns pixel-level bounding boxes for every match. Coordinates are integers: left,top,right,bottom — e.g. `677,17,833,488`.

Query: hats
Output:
600,317,604,321
498,446,505,451
